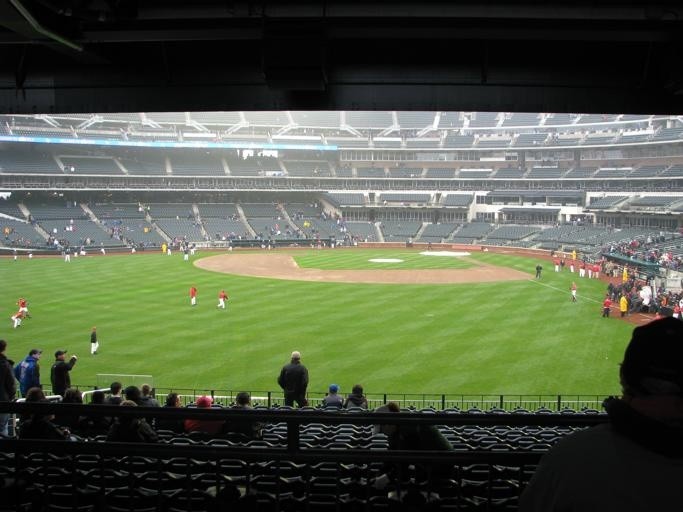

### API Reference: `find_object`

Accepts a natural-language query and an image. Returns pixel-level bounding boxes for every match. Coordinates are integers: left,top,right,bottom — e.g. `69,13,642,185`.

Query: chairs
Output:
0,393,604,512
1,112,682,258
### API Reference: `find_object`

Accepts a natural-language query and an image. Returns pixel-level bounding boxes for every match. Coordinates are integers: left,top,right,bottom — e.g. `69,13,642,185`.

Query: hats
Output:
330,384,339,392
55,350,67,357
291,351,301,359
623,317,683,397
197,397,213,407
30,350,43,356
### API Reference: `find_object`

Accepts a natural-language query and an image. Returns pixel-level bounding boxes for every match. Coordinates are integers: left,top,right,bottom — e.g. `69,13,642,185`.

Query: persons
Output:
0,339,18,437
19,388,84,442
387,402,398,412
11,348,42,397
87,382,161,442
157,393,185,433
189,284,197,306
89,326,98,354
0,114,682,263
15,297,31,317
185,397,215,431
372,417,457,491
50,349,77,396
321,383,368,409
216,289,228,309
515,315,683,509
526,263,682,323
10,310,26,328
276,350,309,406
223,393,256,434
